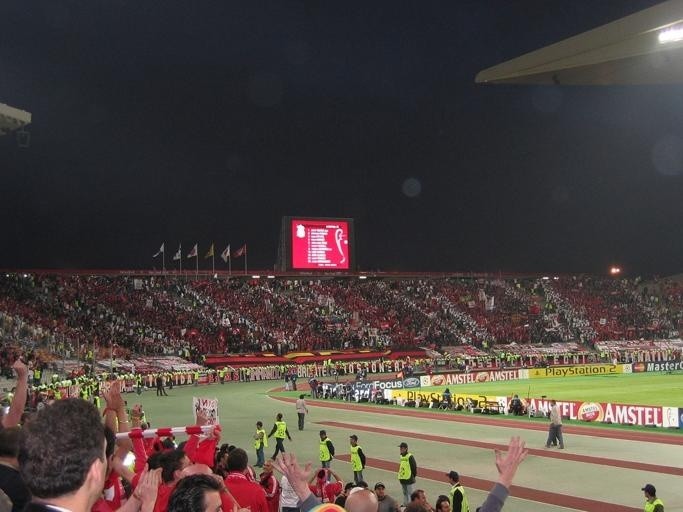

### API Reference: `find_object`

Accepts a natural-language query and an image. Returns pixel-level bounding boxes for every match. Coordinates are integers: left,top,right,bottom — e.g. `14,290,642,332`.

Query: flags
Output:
186,243,197,259
172,242,181,261
233,243,246,257
205,242,214,260
152,241,163,258
220,244,229,263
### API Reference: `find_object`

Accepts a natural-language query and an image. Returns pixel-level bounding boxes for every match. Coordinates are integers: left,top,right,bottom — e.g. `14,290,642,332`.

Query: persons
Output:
0,271,681,512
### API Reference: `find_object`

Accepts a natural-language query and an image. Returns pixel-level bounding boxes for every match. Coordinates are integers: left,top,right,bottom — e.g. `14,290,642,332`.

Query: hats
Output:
446,471,458,480
350,435,357,439
398,443,407,447
642,484,655,496
